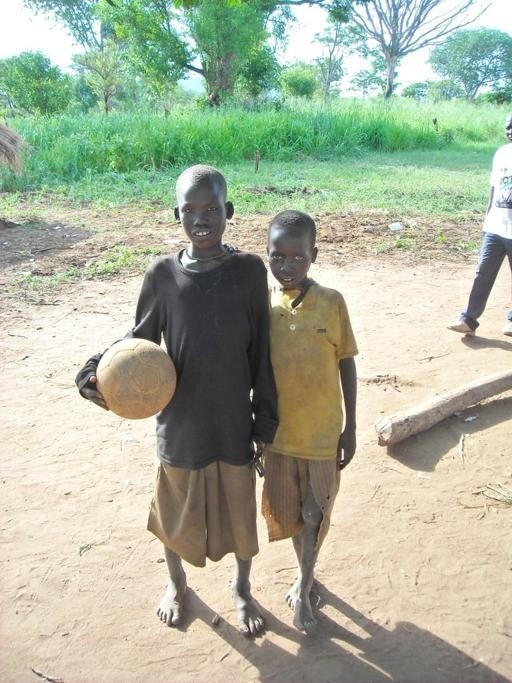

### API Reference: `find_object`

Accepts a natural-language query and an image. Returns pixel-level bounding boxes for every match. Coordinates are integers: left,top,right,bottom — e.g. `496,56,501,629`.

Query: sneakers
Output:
448,322,474,336
504,320,512,335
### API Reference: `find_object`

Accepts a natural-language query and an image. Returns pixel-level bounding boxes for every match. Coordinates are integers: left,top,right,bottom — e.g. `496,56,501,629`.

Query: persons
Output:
262,211,358,632
447,113,512,336
75,164,278,638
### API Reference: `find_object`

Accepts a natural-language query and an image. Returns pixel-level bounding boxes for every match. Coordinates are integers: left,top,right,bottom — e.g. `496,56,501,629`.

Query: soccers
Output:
94,337,177,420
